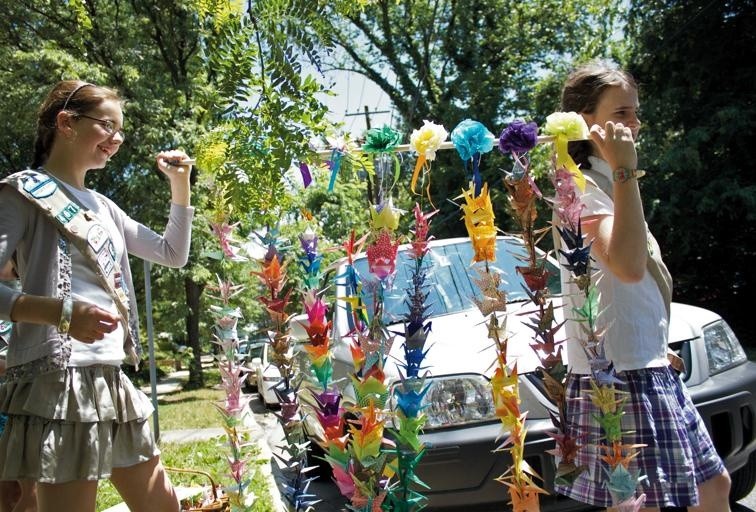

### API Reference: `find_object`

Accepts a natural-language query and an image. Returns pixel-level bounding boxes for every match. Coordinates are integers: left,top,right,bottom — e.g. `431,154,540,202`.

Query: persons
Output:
554,71,731,512
1,79,193,512
1,251,38,512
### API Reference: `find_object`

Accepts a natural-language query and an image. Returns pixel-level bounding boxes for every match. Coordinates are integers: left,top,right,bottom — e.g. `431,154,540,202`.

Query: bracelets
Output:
57,296,73,335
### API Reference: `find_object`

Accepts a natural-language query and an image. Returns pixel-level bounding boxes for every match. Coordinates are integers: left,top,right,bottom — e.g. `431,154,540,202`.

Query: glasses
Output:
78,114,128,140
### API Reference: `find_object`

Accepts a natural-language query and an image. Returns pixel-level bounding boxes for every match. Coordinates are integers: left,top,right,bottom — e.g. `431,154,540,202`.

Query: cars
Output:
292,236,756,512
252,336,293,408
243,343,265,393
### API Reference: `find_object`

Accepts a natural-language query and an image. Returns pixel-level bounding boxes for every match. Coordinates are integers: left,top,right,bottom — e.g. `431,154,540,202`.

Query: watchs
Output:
611,167,645,183
